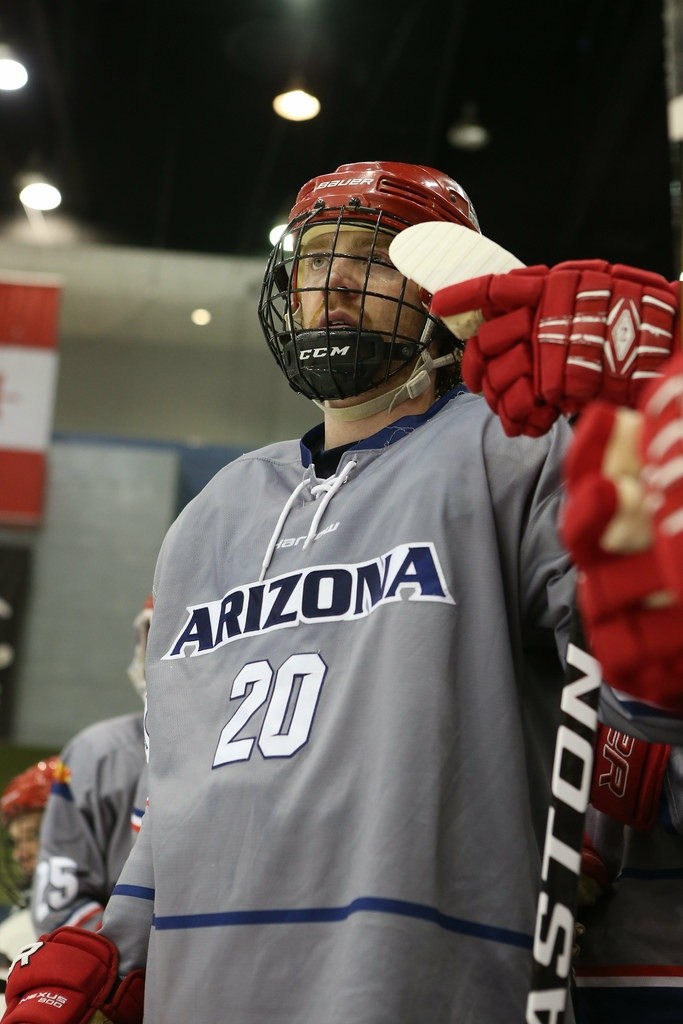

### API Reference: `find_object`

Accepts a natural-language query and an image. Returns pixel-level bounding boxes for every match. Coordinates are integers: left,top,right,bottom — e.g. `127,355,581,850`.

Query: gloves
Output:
429,260,682,439
0,927,145,1024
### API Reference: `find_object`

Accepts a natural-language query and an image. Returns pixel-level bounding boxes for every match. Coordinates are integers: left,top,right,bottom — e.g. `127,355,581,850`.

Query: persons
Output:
30,597,155,945
0,756,64,1016
0,160,682,1024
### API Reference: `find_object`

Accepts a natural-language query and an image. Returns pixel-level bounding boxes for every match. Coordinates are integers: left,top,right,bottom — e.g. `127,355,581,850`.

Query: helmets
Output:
287,161,481,311
134,592,154,627
0,753,60,830
